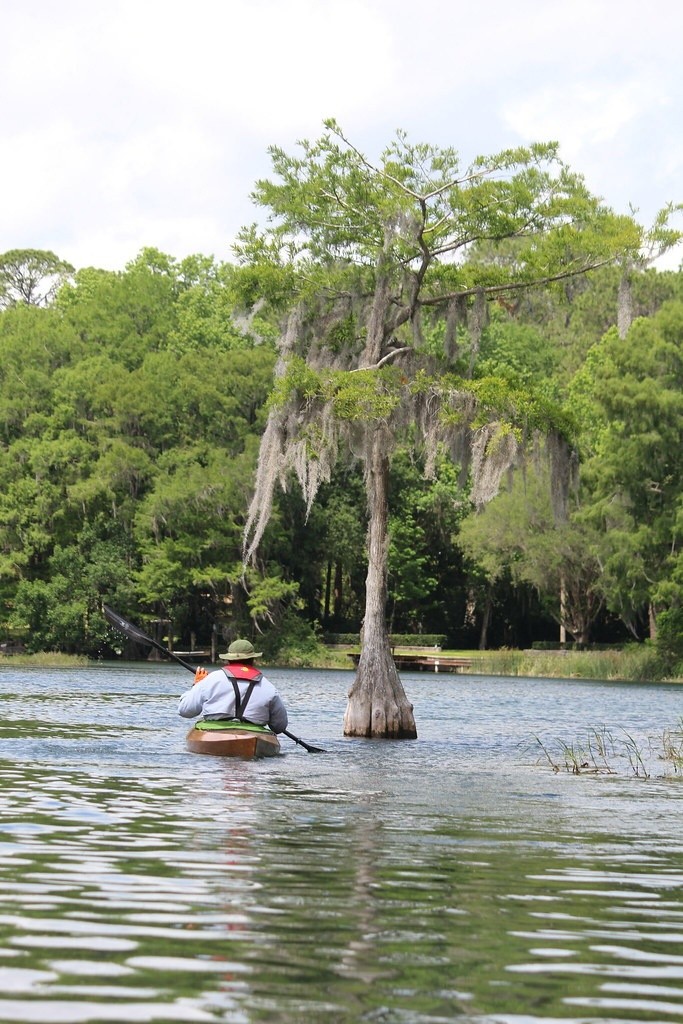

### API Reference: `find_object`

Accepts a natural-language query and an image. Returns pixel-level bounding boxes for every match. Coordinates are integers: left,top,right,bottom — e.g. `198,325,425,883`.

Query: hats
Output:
219,639,263,660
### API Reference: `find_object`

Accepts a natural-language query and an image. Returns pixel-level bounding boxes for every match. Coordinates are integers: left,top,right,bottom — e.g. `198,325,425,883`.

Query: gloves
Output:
194,666,209,684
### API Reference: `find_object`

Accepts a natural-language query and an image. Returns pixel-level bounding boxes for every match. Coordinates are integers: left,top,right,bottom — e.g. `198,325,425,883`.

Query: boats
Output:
185,720,280,760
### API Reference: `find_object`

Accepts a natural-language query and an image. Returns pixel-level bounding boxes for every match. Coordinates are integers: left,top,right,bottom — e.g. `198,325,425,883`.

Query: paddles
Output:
98,601,326,756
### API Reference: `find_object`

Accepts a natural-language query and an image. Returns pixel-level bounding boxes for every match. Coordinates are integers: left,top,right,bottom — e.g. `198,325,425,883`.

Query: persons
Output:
177,639,288,734
